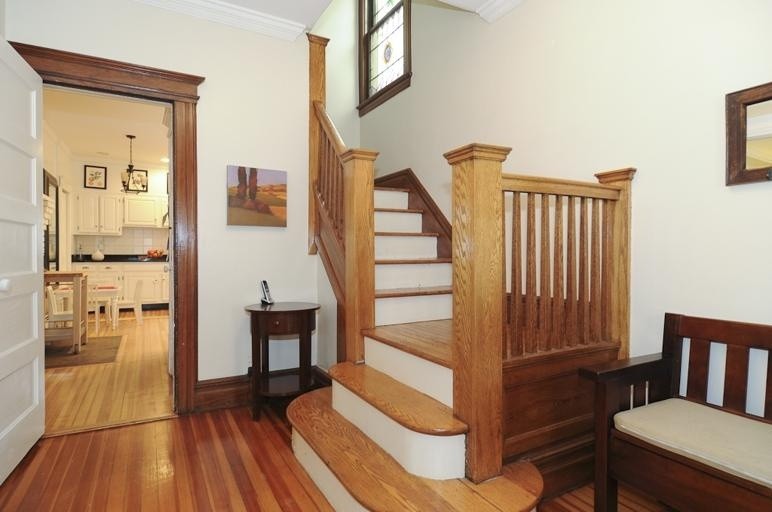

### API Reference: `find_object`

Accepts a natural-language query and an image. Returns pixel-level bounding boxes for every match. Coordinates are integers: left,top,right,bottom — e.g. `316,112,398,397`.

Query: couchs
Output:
577,311,772,512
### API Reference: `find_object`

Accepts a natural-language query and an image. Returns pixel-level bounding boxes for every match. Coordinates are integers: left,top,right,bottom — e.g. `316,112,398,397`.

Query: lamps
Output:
120,134,149,192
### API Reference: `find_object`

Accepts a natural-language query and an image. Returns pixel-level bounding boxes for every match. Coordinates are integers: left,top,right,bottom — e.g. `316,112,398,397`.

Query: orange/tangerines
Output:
148,249,164,257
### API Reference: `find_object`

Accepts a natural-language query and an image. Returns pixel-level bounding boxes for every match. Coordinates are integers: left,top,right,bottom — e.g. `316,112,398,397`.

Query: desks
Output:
243,301,322,421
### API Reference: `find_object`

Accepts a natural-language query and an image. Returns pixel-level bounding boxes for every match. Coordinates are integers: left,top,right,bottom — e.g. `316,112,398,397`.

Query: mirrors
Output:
44,169,60,273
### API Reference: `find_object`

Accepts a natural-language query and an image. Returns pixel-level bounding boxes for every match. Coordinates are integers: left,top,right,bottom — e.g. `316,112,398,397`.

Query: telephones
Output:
260,280,274,305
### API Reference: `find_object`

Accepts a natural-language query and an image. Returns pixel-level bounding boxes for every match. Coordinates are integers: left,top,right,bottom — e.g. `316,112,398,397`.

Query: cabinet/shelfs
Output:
123,194,169,228
71,188,123,236
71,262,170,309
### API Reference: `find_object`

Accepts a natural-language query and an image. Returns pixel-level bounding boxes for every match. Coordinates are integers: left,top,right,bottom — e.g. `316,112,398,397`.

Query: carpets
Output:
45,335,123,368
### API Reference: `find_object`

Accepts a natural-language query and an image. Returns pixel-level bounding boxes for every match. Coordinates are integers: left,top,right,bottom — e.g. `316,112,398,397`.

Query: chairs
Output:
44,272,145,353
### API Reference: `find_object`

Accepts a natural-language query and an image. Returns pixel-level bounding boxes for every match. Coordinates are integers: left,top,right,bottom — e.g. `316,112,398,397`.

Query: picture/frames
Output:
725,81,772,187
127,169,148,192
84,165,107,189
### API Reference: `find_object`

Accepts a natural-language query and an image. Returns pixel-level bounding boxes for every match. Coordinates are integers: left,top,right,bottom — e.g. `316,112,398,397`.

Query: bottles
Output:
91,242,105,261
